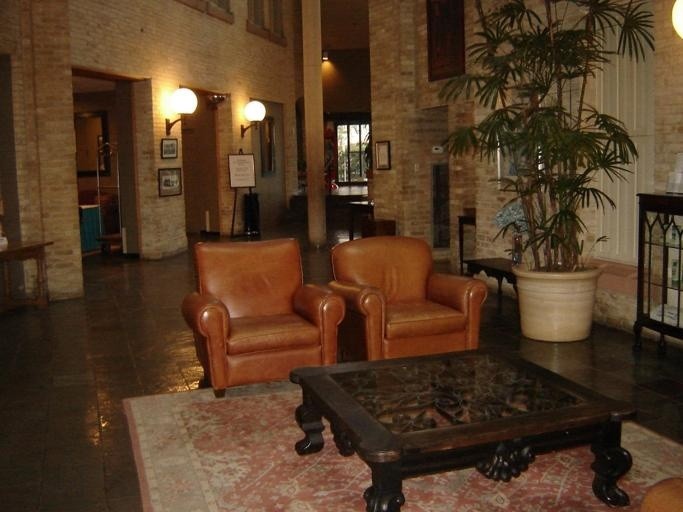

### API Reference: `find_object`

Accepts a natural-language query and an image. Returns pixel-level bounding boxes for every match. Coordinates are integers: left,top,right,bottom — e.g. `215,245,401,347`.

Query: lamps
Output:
322,51,328,61
164,87,197,135
240,100,266,138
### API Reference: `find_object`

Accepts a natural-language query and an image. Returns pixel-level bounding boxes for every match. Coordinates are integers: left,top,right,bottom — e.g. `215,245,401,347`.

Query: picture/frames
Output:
376,141,391,171
75,109,112,178
259,116,276,178
161,138,178,159
158,168,182,196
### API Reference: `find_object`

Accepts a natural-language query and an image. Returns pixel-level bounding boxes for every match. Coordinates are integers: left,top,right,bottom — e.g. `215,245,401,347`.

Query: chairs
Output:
182,236,347,398
329,235,488,361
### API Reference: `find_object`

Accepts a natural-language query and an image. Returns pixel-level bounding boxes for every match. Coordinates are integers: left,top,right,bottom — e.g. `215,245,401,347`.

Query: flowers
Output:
495,203,528,231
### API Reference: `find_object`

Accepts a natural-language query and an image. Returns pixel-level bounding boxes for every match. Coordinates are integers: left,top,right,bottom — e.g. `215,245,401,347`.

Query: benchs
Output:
464,258,519,298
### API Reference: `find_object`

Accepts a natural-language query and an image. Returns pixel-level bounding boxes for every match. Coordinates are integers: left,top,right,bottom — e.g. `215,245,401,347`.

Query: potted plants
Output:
436,0,656,342
364,130,374,199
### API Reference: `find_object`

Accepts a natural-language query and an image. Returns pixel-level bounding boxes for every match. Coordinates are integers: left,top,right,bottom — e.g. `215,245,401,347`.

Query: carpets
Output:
123,360,682,511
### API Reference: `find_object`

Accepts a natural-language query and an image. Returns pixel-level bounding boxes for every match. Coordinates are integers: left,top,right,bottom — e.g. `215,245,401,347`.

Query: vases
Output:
512,232,523,263
462,208,475,215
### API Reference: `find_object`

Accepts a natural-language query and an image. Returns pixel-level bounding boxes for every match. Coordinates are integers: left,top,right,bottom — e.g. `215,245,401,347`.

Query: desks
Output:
347,200,374,241
457,216,475,274
0,239,54,316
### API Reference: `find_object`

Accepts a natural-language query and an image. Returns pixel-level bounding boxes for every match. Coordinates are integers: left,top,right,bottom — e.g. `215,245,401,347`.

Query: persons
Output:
164,143,175,154
162,174,179,188
